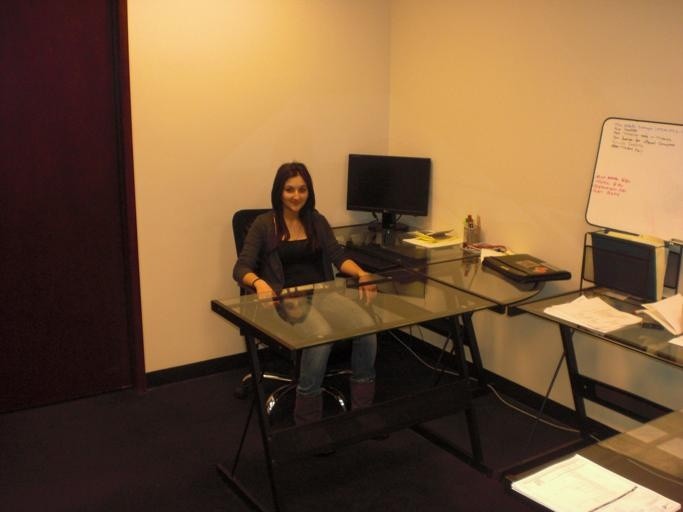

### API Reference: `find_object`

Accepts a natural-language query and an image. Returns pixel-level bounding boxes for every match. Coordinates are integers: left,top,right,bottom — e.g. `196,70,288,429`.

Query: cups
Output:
462,225,480,248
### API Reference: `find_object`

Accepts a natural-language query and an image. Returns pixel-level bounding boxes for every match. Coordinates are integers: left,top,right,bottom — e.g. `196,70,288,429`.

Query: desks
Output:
512,285,683,443
329,221,484,390
496,407,683,512
409,259,596,480
213,269,501,512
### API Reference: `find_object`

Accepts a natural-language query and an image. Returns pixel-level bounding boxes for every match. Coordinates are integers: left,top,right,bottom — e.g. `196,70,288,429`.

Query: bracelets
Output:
252,278,261,289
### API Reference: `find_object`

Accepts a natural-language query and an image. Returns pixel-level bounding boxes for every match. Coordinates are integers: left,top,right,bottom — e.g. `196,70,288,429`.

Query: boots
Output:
293,397,336,457
348,374,389,440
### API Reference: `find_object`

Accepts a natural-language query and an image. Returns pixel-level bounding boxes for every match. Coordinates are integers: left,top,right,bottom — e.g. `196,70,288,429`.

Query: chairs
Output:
230,208,355,422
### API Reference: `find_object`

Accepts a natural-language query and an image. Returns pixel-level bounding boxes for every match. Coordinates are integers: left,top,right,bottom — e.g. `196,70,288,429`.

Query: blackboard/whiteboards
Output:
585,117,683,246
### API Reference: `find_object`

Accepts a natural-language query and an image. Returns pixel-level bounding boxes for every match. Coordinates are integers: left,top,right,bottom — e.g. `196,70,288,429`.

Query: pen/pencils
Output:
464,215,480,243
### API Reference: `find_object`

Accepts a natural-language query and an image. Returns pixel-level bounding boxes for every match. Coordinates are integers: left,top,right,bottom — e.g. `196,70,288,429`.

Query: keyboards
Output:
342,247,400,270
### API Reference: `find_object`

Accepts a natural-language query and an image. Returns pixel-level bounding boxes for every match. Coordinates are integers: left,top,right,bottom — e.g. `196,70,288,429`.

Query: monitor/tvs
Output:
346,153,430,232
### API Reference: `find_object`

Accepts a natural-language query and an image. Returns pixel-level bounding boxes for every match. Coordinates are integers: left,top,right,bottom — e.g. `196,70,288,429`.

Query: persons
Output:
234,162,388,457
272,283,314,325
